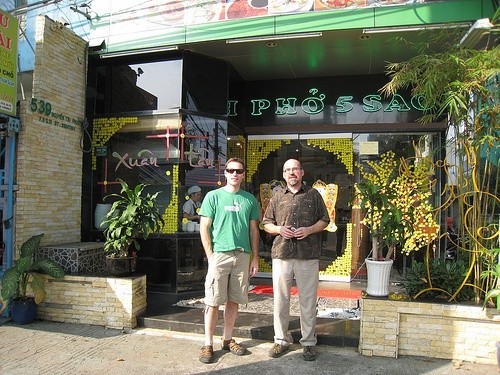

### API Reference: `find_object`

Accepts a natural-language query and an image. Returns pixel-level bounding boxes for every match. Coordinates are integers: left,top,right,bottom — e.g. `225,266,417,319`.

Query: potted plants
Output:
97,178,162,277
1,234,65,325
94,193,112,229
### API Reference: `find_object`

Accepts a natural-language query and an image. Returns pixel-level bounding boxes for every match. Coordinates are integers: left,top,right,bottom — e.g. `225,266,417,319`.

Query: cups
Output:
186,222,194,232
194,224,200,232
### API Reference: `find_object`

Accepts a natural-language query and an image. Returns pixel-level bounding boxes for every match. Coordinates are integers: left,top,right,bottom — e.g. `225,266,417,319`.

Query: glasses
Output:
225,168,244,174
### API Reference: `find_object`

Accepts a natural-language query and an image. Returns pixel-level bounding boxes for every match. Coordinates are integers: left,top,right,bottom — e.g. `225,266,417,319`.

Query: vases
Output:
364,259,392,296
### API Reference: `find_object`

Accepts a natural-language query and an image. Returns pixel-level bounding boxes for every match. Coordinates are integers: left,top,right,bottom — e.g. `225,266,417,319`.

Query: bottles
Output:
182,218,188,232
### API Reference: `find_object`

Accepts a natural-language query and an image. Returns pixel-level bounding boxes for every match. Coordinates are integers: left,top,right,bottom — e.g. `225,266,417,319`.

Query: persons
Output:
335,209,352,256
262,158,330,361
198,158,259,362
182,186,202,231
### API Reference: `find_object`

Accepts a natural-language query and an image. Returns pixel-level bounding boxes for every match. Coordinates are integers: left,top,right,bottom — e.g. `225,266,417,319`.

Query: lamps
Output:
363,21,474,34
224,32,324,44
99,45,178,58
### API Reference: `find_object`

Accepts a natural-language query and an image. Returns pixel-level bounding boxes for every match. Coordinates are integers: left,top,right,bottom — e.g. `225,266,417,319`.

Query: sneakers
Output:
220,337,246,356
199,344,214,363
302,345,319,361
269,343,289,358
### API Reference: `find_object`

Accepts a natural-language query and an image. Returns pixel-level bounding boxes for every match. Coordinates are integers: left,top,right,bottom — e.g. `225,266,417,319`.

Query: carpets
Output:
173,284,360,321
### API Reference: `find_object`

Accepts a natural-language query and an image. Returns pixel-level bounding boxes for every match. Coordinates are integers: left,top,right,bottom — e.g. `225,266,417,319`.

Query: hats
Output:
187,185,201,195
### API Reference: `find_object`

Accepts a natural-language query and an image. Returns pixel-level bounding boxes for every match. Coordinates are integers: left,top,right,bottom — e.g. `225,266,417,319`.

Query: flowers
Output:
350,153,438,260
